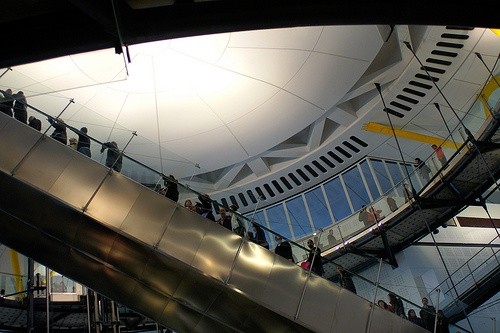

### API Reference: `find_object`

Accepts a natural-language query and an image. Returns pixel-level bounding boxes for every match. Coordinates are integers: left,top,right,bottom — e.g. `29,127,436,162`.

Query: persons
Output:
432,144,447,166
184,193,269,250
163,174,179,202
306,239,324,277
28,116,41,131
387,195,398,212
359,205,382,227
69,127,91,158
327,229,336,245
0,89,27,124
403,183,413,202
47,117,67,145
337,266,356,293
414,158,430,182
275,236,295,263
100,141,122,173
378,293,450,333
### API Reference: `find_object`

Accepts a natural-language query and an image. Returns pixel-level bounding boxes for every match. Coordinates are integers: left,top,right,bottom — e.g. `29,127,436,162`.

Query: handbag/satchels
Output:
301,262,310,271
